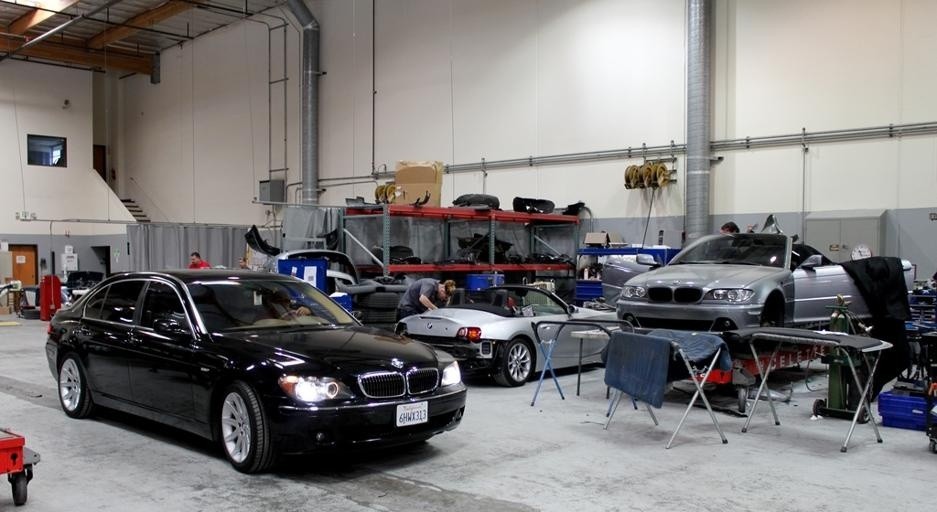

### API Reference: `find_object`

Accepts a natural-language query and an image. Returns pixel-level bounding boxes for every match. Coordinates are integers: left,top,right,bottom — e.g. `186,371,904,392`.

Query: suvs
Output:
616,230,915,349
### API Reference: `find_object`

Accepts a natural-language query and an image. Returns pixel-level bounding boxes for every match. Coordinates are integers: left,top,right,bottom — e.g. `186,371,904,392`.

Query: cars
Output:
43,268,466,474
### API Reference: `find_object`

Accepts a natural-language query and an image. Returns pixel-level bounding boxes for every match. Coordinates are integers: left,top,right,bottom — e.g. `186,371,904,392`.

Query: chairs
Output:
391,160,443,207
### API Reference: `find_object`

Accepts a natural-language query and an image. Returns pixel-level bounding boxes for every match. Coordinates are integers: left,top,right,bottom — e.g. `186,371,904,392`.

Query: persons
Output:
396,277,456,323
256,290,312,321
712,221,740,234
238,257,250,270
188,252,211,269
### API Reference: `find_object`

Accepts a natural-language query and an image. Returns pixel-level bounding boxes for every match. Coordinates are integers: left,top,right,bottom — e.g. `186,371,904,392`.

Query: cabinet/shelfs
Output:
342,206,580,290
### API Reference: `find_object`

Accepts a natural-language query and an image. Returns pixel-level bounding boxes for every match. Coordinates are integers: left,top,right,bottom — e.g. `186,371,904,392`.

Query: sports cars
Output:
394,284,622,384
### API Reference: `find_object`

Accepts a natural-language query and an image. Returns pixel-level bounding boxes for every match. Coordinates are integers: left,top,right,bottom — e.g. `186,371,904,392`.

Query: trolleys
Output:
0,425,40,509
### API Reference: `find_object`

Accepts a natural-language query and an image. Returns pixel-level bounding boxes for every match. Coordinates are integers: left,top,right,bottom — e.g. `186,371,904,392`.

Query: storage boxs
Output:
877,391,928,429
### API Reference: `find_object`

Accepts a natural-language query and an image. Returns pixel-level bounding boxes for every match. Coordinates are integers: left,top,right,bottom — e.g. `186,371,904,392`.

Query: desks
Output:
573,327,623,400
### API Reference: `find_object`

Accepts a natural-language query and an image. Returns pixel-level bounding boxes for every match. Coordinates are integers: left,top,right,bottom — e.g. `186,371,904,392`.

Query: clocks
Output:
849,245,872,260
